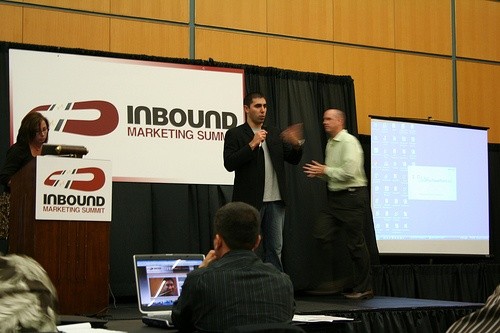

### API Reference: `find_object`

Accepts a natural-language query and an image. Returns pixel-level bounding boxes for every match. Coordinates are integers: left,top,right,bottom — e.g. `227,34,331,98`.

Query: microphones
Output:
258,124,268,150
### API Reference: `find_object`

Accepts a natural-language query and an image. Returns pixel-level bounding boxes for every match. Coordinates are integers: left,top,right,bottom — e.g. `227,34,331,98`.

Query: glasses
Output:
36,128,48,134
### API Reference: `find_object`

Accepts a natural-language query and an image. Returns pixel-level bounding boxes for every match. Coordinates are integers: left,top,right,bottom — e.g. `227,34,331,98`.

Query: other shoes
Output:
343,288,373,301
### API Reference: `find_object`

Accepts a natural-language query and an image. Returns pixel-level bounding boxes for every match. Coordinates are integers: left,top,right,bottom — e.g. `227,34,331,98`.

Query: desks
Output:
105,320,309,333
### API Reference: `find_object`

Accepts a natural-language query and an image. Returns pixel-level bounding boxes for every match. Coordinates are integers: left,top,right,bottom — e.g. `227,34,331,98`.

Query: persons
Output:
170,201,295,333
161,278,175,296
224,91,306,276
302,108,375,300
0,112,49,255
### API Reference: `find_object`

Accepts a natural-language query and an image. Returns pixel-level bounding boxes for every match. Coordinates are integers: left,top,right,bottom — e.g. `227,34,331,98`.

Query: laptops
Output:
40,142,84,158
132,254,206,329
